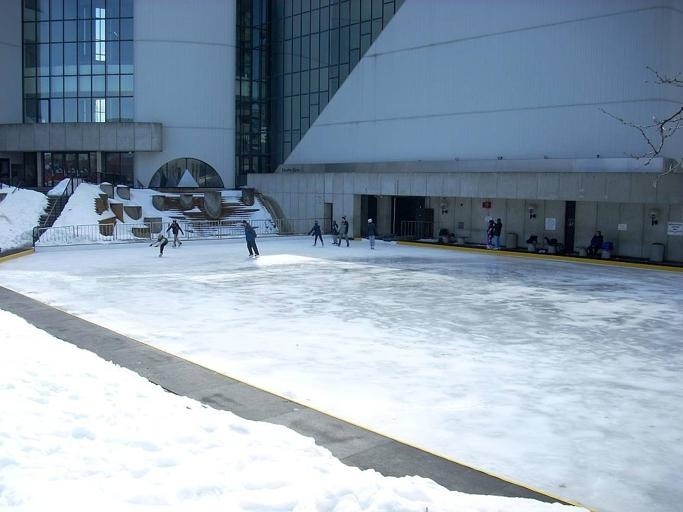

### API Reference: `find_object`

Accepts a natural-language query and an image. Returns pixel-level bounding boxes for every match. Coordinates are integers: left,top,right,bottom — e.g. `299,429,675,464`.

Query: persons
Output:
586,230,603,258
149,233,168,257
308,220,324,246
331,220,340,246
485,218,502,250
166,219,184,247
337,214,351,246
242,220,260,256
367,218,376,250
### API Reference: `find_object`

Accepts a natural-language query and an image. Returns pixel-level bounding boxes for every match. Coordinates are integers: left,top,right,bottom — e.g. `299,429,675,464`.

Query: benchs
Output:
448,236,472,244
527,242,561,254
574,245,614,259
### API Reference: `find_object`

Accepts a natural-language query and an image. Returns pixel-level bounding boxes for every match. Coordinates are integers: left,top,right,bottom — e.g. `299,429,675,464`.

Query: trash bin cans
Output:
651,243,664,262
506,233,517,249
